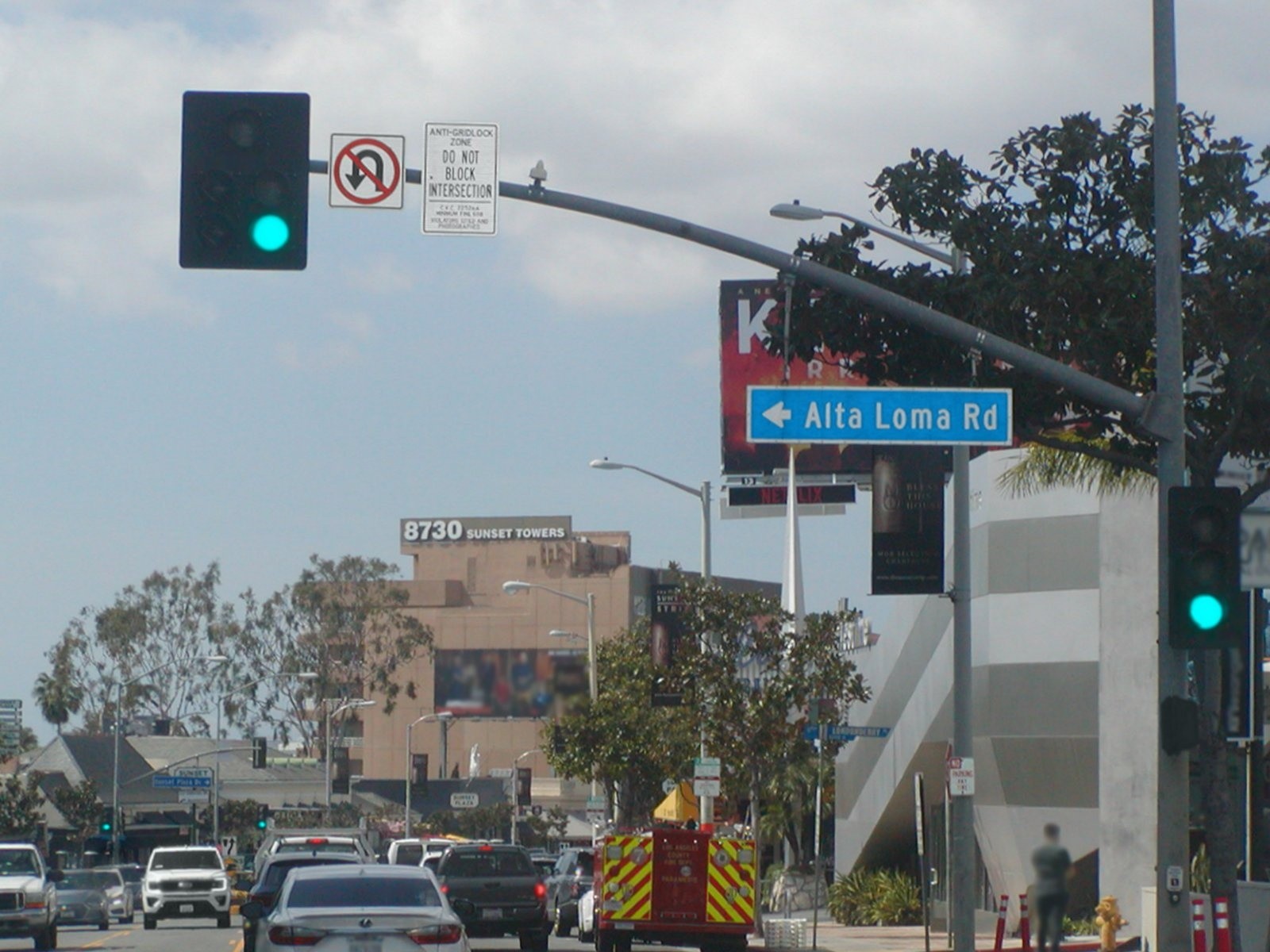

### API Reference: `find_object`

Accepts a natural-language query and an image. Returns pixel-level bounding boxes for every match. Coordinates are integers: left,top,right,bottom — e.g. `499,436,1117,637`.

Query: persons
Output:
1030,823,1074,952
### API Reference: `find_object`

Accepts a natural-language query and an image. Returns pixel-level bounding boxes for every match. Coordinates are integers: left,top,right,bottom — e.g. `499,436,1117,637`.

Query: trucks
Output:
592,825,758,952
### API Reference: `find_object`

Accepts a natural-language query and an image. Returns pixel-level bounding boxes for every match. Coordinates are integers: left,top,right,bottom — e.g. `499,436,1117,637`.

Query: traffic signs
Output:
802,723,893,742
152,776,215,789
744,383,1014,449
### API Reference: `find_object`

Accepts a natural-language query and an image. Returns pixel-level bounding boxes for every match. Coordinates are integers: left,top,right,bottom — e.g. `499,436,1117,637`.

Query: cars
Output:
576,887,597,944
256,864,472,952
52,861,148,931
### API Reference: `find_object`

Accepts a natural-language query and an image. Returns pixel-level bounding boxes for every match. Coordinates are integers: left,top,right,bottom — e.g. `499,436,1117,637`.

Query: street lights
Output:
512,746,550,846
110,656,229,864
213,671,317,848
402,710,455,838
502,582,600,705
587,456,716,831
324,697,377,824
772,200,977,951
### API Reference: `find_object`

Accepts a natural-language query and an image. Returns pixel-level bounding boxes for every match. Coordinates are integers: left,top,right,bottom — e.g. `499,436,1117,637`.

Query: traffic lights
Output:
257,803,268,831
1165,483,1243,653
175,89,311,272
98,818,111,840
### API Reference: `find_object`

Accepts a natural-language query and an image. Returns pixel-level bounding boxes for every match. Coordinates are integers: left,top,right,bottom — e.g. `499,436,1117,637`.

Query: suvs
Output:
544,846,594,939
387,839,560,952
139,845,232,930
0,842,58,951
237,826,381,952
